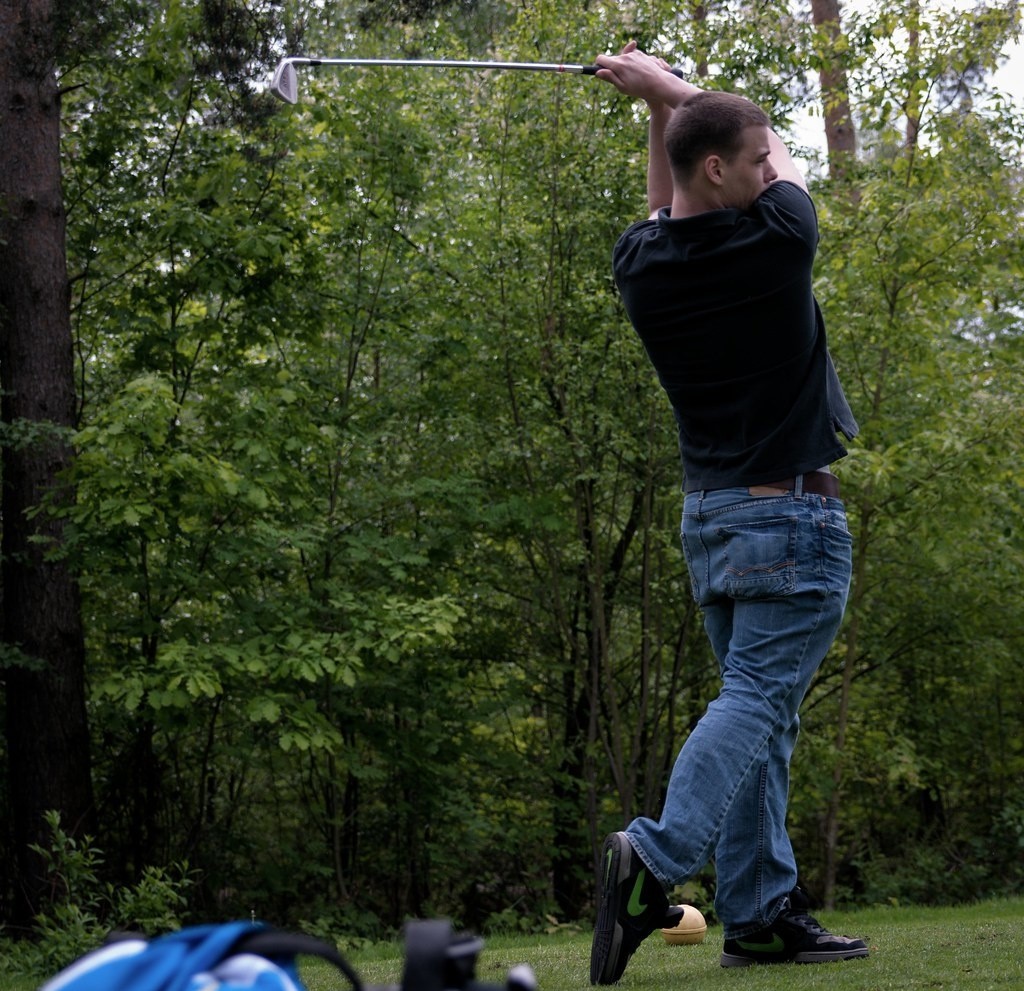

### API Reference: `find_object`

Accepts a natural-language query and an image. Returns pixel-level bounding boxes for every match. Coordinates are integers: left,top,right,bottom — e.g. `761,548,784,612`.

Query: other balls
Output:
663,901,707,947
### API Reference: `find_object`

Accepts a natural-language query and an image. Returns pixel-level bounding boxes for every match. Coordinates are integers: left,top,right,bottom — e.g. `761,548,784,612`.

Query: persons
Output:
589,42,870,985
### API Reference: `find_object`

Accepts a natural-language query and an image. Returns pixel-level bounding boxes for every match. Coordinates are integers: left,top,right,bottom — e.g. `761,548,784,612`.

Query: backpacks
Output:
42,917,374,990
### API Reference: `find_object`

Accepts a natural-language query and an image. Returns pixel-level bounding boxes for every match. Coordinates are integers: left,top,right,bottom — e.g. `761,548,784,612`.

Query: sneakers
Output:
589,833,684,986
722,909,867,966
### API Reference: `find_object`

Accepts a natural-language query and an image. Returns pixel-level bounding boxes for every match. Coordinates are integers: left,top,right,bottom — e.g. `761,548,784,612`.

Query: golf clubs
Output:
270,55,685,103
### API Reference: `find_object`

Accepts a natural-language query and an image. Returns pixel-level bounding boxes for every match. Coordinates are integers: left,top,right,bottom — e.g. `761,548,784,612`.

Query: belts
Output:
762,466,842,500
